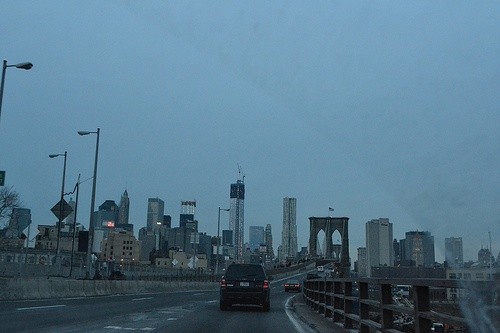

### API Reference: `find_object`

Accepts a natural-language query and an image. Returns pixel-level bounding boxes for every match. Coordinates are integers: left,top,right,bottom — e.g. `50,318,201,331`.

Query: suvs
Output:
220,264,271,312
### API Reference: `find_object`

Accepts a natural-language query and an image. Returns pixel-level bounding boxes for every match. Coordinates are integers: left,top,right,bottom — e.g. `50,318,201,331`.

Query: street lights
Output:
77,128,101,279
49,151,68,265
215,207,230,282
0,60,33,122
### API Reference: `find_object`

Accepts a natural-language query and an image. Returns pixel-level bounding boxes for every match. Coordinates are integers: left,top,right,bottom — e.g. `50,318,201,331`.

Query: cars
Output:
285,280,302,293
